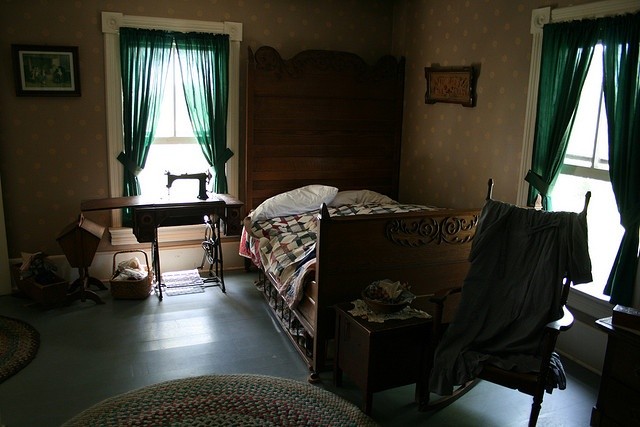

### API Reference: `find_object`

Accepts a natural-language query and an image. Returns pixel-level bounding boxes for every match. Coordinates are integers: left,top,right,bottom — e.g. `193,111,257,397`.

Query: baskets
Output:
109,249,153,300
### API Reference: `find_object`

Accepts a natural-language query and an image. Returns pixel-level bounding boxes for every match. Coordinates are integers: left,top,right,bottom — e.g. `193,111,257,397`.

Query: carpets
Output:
0,313,42,387
57,374,382,427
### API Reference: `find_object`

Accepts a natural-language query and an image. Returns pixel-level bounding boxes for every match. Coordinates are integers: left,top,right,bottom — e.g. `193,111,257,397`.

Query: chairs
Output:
415,179,590,427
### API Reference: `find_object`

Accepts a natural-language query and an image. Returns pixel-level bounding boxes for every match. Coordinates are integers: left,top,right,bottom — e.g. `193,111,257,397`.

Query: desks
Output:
80,193,244,302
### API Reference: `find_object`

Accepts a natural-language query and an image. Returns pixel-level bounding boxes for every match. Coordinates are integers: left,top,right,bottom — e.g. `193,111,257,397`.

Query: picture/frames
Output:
423,67,479,106
12,43,81,98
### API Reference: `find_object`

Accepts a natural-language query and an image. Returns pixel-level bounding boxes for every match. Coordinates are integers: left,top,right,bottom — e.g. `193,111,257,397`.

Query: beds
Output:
239,184,531,384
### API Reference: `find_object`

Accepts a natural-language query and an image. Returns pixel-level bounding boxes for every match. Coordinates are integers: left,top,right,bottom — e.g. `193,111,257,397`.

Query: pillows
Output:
248,185,336,217
340,189,396,206
